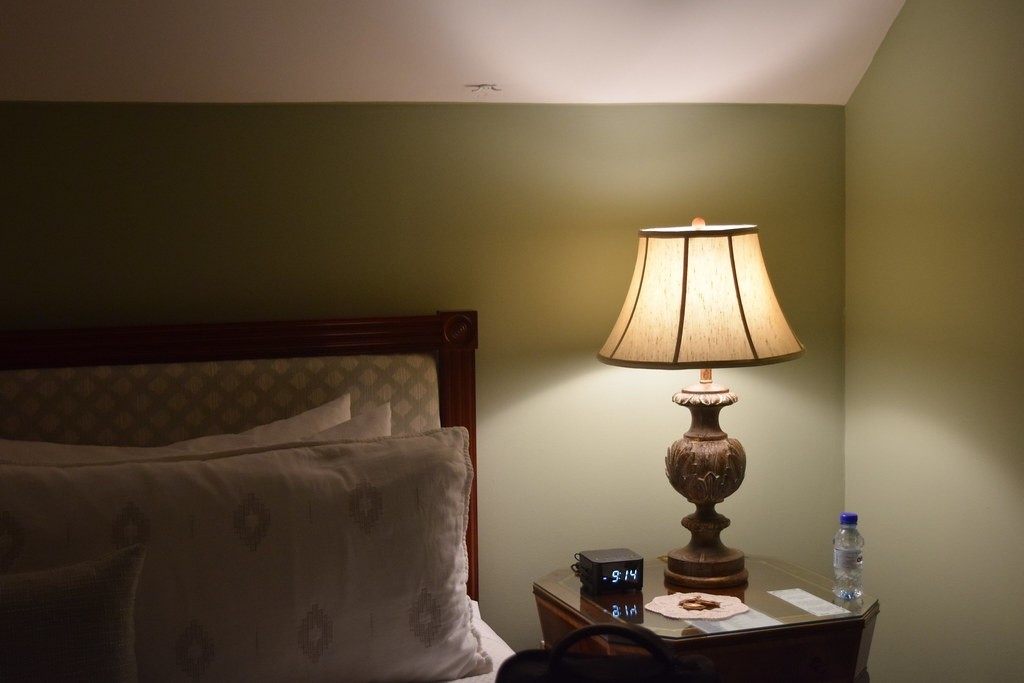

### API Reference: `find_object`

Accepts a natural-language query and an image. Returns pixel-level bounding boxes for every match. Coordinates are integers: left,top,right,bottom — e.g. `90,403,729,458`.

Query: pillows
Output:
0,541,150,683
0,427,494,683
0,391,393,466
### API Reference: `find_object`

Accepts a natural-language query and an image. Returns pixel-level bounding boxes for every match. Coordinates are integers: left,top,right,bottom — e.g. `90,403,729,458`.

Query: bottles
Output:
832,512,864,599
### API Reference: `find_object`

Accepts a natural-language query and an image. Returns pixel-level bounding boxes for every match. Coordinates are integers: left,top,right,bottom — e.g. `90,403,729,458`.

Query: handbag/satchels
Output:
495,623,718,683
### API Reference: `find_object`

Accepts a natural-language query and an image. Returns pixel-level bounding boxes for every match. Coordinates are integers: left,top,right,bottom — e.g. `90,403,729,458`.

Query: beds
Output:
0,310,478,601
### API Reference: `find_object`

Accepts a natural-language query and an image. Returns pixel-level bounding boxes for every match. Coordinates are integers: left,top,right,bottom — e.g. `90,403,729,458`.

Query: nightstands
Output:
532,553,880,683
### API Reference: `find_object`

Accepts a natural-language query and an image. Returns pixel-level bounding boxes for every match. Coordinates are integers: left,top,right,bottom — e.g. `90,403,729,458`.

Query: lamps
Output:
597,218,806,589
